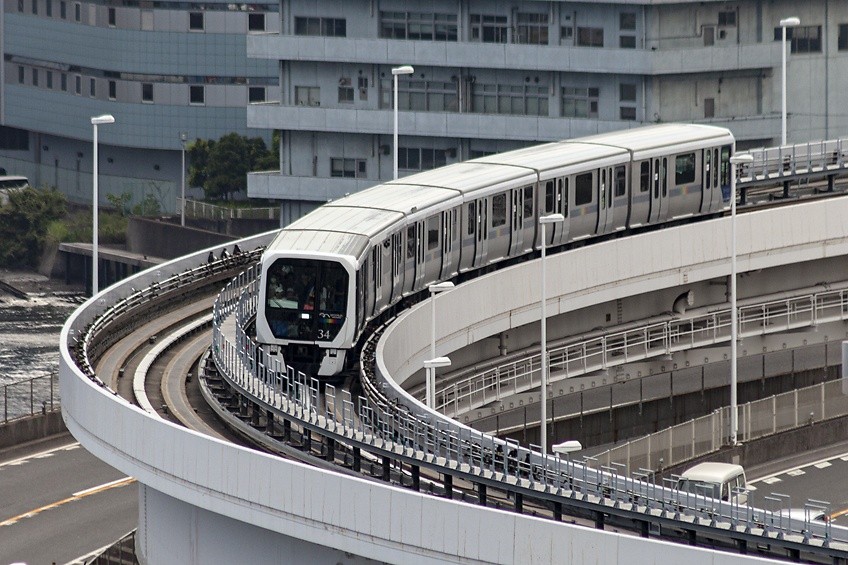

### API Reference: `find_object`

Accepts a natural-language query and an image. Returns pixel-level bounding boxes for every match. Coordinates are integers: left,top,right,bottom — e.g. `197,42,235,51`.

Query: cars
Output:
775,508,830,524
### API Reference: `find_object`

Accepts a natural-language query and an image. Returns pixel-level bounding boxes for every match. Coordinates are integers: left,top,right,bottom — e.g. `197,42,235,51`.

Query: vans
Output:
673,462,748,508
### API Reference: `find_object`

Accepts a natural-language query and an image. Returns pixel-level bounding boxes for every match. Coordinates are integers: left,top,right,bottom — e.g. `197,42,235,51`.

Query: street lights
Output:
91,114,115,294
729,153,753,445
429,282,454,410
392,66,415,179
779,16,800,146
539,213,565,455
423,358,452,408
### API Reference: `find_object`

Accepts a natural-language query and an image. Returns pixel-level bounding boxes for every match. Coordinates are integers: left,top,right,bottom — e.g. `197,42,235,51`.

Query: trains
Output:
255,122,735,389
